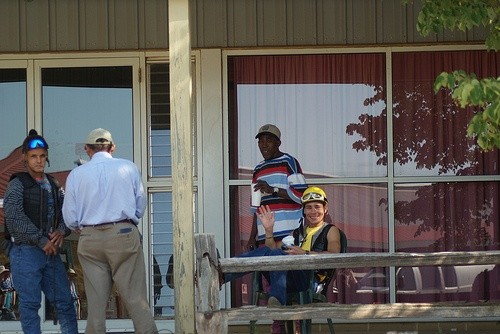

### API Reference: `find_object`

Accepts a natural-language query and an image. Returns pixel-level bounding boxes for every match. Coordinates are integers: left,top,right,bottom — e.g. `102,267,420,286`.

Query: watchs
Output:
273,186,279,198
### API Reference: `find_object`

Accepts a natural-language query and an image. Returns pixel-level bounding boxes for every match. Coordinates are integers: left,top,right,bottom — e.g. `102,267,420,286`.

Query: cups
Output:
251,183,262,206
282,236,295,246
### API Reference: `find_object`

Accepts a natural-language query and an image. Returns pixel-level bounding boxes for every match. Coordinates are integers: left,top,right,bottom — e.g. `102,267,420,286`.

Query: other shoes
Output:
268,297,282,307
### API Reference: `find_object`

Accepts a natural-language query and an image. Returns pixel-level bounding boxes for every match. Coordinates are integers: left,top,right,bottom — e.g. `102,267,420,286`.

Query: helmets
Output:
303,187,328,205
22,130,48,152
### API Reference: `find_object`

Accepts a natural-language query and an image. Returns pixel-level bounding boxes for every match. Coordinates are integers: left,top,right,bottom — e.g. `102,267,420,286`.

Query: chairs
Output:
249,229,348,334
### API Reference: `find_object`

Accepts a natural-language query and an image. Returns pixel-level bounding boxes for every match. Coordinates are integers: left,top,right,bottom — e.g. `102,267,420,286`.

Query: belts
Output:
84,219,132,227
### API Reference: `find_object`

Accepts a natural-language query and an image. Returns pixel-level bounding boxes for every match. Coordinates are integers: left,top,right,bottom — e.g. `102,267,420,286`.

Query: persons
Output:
2,133,79,334
217,187,341,307
248,123,309,306
62,128,158,334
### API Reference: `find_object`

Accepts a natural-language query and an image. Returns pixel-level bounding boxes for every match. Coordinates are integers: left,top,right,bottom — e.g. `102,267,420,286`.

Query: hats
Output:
84,128,111,149
255,124,281,141
68,269,77,276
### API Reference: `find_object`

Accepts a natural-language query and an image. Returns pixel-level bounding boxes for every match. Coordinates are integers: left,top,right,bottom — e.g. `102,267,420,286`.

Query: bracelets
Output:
266,236,274,239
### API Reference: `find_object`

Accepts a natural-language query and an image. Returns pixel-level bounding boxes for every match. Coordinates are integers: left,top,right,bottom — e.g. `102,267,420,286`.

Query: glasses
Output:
302,192,325,201
